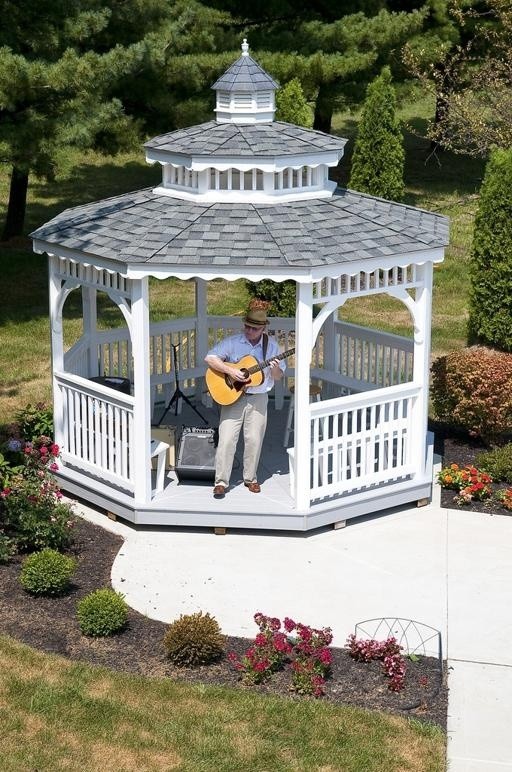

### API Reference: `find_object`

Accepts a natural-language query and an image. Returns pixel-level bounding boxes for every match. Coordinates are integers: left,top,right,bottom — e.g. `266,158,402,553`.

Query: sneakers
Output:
214,486,225,495
245,483,260,493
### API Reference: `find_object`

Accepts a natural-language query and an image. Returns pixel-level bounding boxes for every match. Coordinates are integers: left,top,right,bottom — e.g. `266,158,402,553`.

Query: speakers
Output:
175,424,219,486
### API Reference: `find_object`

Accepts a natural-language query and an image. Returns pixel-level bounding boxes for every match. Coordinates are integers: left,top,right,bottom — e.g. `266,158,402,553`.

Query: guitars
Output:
204,348,295,406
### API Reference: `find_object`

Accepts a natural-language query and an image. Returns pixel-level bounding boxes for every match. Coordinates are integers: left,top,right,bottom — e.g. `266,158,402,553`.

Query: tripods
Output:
155,343,209,427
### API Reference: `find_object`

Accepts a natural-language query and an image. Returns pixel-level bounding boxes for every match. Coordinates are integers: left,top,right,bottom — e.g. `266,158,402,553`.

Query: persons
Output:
204,308,286,495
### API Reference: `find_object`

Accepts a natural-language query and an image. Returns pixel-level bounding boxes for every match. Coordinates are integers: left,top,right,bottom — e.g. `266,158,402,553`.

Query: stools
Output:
283,384,327,447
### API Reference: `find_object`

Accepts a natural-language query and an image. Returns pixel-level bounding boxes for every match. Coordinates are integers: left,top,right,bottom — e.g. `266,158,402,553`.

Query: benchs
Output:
114,367,211,416
74,410,170,497
272,364,408,469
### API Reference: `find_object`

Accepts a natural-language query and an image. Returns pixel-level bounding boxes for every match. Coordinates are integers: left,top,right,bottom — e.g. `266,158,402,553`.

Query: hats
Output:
242,310,270,327
